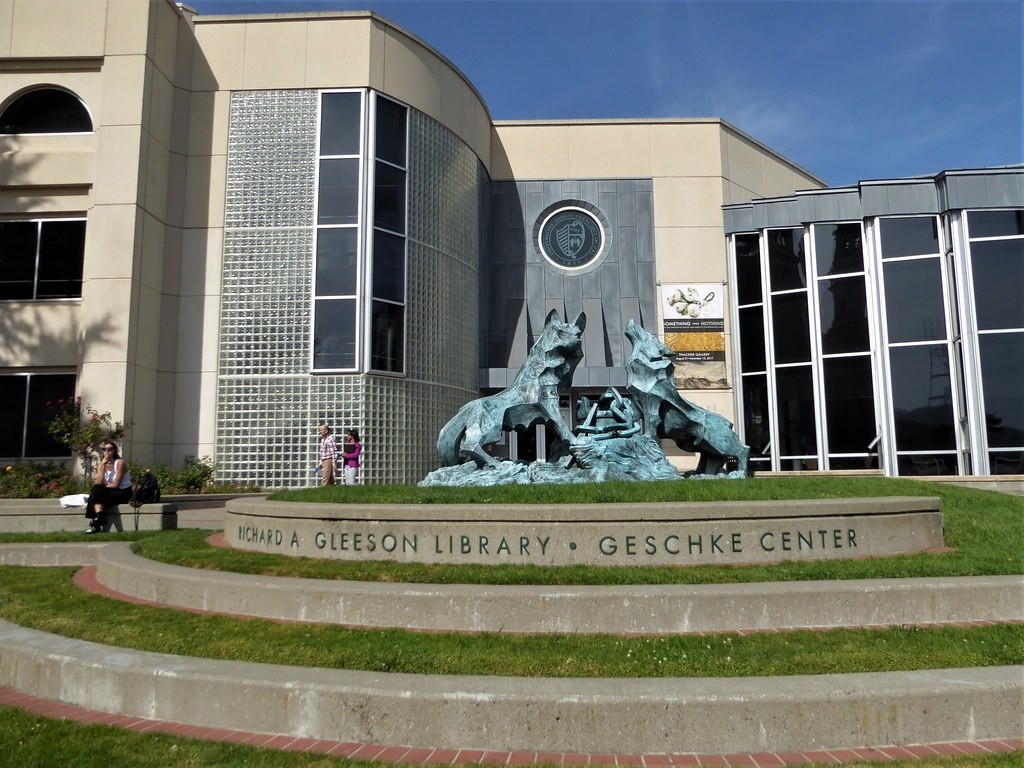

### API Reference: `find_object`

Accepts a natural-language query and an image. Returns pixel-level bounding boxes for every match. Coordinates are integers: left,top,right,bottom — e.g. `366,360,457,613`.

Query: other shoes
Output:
85,520,102,533
95,511,109,525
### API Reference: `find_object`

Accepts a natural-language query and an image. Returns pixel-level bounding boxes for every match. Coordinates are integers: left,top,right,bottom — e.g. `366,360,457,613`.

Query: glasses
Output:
102,447,113,450
348,434,351,435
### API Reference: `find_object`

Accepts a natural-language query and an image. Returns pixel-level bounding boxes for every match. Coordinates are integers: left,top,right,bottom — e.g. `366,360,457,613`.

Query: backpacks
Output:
131,472,160,530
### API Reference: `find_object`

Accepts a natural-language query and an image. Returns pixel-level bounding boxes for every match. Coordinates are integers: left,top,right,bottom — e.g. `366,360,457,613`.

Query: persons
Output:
318,425,339,486
342,430,361,485
82,442,133,534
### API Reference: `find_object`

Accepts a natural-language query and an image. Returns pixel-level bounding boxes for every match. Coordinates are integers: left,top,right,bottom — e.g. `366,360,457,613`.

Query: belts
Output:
322,457,332,461
